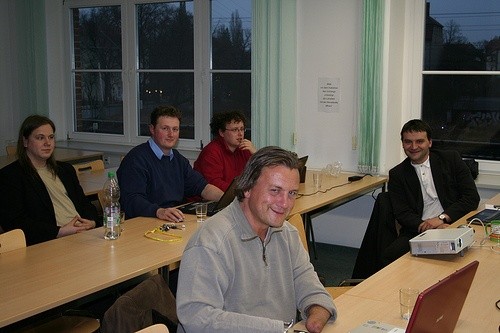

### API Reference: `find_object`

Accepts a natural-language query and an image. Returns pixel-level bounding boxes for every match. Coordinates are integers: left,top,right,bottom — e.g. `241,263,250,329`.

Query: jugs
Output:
327,161,344,177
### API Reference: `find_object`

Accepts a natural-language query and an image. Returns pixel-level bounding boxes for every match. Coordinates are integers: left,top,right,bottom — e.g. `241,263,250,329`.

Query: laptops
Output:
466,209,500,225
175,176,239,215
347,261,478,332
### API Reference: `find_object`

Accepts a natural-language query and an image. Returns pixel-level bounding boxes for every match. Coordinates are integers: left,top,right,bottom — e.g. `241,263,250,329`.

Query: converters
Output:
347,175,363,180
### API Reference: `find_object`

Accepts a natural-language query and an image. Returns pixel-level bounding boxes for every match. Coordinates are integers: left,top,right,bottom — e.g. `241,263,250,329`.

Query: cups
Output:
119,211,125,233
399,288,420,320
313,172,322,187
196,204,207,222
485,220,500,243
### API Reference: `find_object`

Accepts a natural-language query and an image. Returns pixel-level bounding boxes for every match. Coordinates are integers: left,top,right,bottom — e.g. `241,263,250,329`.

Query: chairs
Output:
135,324,170,333
352,192,401,277
0,229,101,333
72,160,105,171
97,190,115,214
104,273,179,333
6,144,17,155
285,213,367,299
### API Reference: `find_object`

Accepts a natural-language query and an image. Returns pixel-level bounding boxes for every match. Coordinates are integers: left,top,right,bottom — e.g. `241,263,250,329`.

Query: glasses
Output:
224,128,246,132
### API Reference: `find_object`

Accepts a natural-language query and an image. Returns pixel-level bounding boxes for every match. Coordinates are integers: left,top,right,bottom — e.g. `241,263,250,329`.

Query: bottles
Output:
101,171,121,239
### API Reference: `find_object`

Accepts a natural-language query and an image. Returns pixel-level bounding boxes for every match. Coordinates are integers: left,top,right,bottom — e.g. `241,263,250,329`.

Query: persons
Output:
185,110,258,203
0,114,107,246
382,120,481,268
116,106,222,224
175,145,338,332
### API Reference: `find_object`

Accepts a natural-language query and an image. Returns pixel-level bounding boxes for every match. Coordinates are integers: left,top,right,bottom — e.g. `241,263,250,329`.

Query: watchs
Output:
438,214,448,224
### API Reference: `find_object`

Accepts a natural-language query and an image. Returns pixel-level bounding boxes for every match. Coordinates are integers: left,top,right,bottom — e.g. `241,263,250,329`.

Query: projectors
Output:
408,227,476,257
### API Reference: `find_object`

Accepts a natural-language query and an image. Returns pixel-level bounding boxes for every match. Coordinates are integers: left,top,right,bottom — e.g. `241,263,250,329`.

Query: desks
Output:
0,168,391,330
289,191,500,333
0,147,104,169
76,166,119,195
